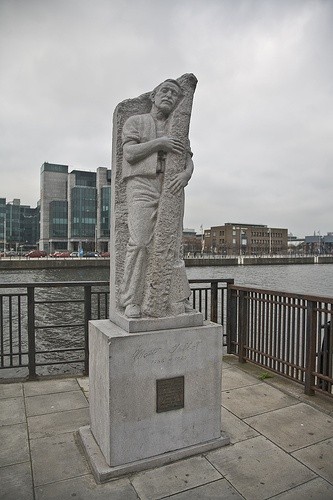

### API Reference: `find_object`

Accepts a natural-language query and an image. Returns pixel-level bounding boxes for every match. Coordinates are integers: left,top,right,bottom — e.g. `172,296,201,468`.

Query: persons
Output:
121,79,198,319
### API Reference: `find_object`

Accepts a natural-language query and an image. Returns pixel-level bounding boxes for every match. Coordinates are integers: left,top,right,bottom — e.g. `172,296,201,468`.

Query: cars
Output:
52,250,70,257
102,252,109,257
70,252,79,257
83,251,100,257
26,250,47,257
0,250,28,257
